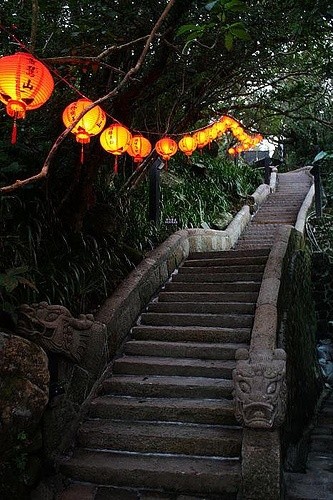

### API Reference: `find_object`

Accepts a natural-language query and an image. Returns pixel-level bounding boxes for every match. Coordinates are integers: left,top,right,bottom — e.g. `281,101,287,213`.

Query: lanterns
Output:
178,135,198,158
126,132,151,172
0,52,53,144
99,122,133,173
61,97,106,164
156,135,178,170
194,114,262,155
